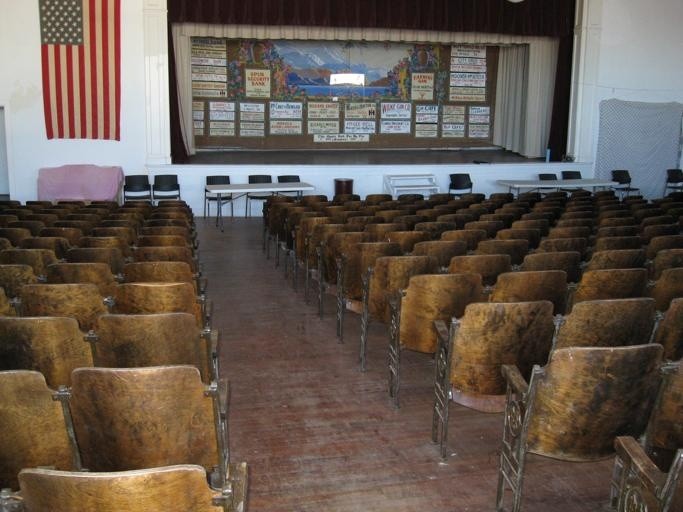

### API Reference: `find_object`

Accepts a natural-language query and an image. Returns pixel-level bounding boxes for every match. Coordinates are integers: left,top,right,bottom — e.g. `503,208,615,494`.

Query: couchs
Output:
38,165,123,202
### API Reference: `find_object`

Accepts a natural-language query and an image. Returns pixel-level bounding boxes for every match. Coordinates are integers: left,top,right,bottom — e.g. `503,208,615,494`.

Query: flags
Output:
37,0,122,144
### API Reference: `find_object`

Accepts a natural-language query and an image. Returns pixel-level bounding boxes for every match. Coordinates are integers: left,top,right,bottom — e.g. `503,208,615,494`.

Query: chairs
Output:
204,176,233,219
245,174,272,218
153,175,180,205
124,176,153,202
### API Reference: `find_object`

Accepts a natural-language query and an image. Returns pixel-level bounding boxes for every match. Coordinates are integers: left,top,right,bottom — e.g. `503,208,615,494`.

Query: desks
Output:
205,182,314,232
